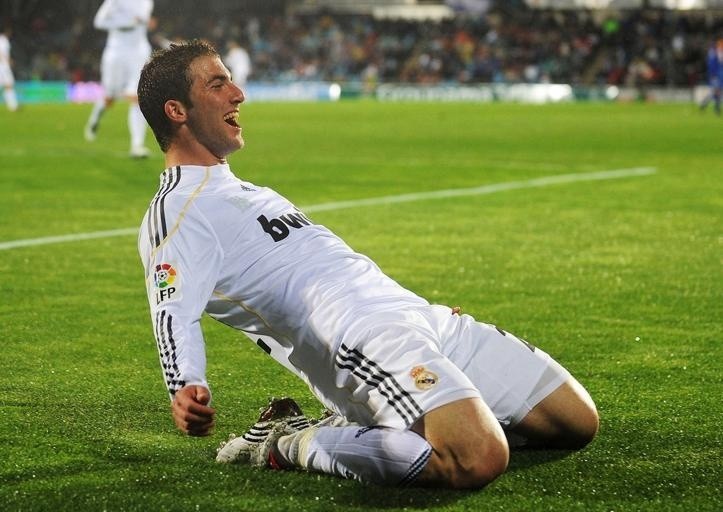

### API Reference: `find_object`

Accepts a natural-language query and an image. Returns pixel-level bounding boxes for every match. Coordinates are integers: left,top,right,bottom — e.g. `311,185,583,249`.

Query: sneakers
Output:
307,409,362,430
215,394,312,470
83,122,98,142
128,146,151,159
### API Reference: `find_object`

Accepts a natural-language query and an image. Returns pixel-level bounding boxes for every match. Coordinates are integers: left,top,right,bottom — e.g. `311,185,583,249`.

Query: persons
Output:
697,36,721,113
149,0,722,88
83,0,156,157
0,28,23,110
1,0,108,83
134,37,600,492
